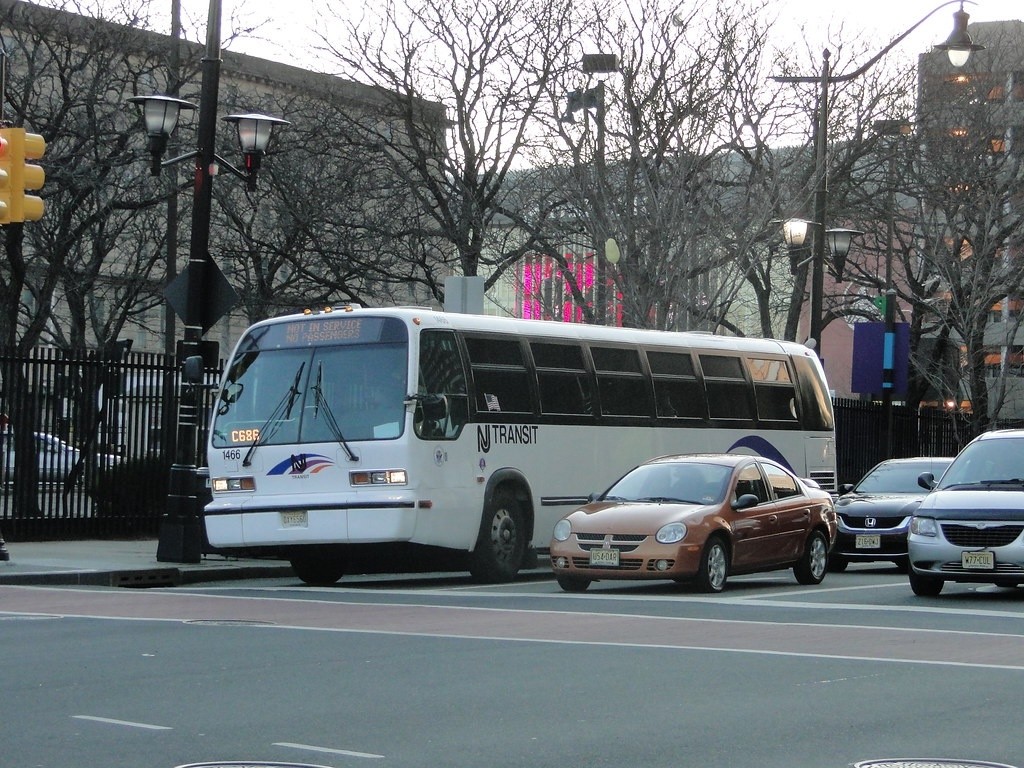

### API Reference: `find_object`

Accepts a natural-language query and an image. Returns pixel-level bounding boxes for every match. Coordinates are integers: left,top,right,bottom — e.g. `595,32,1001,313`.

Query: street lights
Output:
872,116,913,321
127,94,290,565
808,1,986,359
769,215,865,364
566,50,644,332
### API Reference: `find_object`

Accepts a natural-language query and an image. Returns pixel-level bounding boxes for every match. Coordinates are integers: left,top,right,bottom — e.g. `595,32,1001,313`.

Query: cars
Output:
0,432,125,481
832,455,954,572
549,451,837,594
907,428,1024,598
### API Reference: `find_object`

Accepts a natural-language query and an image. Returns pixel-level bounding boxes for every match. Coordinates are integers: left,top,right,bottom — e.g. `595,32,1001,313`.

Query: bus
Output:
201,300,829,587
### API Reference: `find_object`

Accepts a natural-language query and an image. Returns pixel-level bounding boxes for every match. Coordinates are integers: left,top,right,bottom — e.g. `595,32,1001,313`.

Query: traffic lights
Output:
0,125,46,224
